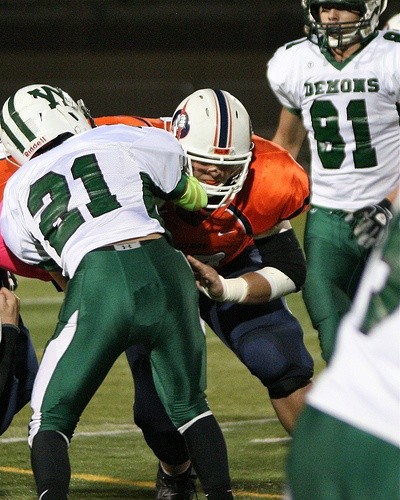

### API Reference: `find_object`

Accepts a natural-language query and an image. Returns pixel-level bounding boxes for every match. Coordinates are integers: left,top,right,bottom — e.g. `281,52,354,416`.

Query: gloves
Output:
343,195,393,251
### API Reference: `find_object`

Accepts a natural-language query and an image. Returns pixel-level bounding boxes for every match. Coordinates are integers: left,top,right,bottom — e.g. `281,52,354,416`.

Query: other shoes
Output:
156,464,196,500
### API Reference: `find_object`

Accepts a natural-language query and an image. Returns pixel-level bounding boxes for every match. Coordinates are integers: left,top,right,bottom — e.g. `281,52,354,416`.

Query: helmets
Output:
168,88,255,208
301,0,388,48
0,85,97,166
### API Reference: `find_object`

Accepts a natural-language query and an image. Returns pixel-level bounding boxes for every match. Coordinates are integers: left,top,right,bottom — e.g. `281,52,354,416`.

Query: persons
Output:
87,88,316,500
1,84,234,500
285,202,398,500
266,0,399,366
0,286,24,435
